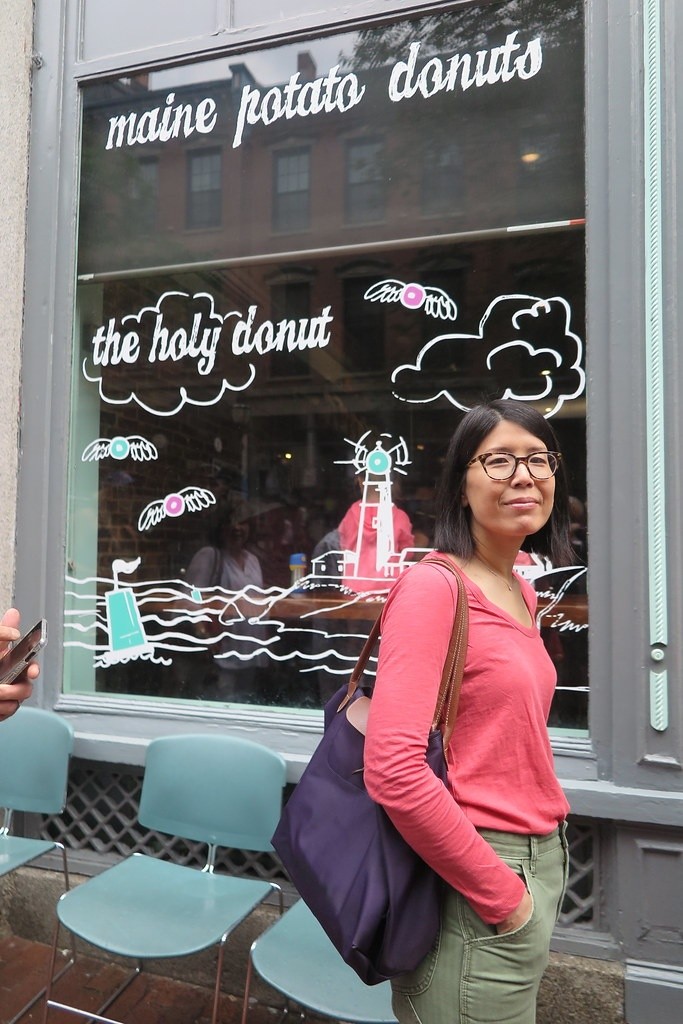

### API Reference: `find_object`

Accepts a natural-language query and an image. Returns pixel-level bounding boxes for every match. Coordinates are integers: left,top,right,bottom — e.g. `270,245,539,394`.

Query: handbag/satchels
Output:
169,548,223,659
270,559,469,990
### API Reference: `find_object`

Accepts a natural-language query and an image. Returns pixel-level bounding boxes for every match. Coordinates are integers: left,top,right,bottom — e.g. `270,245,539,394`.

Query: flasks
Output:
289,553,309,598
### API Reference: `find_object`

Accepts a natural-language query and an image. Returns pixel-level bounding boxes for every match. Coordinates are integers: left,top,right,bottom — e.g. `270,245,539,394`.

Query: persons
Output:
339,470,429,657
308,528,430,700
513,551,564,665
178,503,270,702
0,608,40,720
363,399,571,1024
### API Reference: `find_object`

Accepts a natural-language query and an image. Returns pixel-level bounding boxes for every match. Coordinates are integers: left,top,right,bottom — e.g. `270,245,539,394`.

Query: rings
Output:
18,700,22,706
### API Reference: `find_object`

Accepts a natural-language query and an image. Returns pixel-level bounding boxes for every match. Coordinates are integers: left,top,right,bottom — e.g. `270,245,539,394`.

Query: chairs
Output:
0,706,400,1024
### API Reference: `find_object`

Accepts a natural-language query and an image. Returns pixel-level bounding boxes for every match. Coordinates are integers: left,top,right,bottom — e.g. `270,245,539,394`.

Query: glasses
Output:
466,451,563,481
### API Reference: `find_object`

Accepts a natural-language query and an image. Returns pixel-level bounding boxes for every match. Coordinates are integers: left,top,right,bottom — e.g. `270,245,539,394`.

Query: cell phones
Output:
0,618,49,685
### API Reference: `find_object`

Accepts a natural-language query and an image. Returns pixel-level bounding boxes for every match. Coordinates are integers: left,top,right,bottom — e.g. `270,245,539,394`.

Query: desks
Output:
141,589,587,703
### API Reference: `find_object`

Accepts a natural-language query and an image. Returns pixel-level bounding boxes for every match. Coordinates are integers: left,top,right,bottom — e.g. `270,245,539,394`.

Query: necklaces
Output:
479,561,515,591
